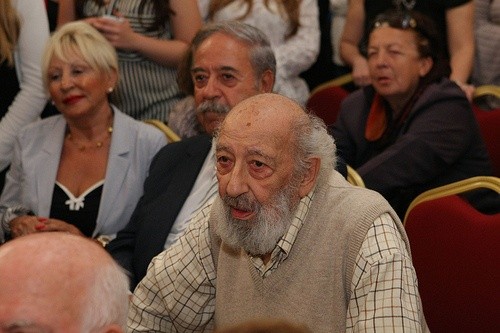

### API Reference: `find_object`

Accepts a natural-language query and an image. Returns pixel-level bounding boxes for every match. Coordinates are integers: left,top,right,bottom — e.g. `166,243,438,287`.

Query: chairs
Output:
143,73,500,333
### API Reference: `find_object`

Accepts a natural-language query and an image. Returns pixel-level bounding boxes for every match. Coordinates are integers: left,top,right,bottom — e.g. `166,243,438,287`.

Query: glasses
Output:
372,12,437,46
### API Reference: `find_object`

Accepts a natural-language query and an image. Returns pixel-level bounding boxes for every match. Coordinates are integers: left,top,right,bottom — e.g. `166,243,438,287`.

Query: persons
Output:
0,0,500,333
0,20,169,245
127,93,430,333
329,12,500,218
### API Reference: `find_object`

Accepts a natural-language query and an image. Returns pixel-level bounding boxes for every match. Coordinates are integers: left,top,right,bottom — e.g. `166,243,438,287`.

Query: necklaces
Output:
64,127,114,151
